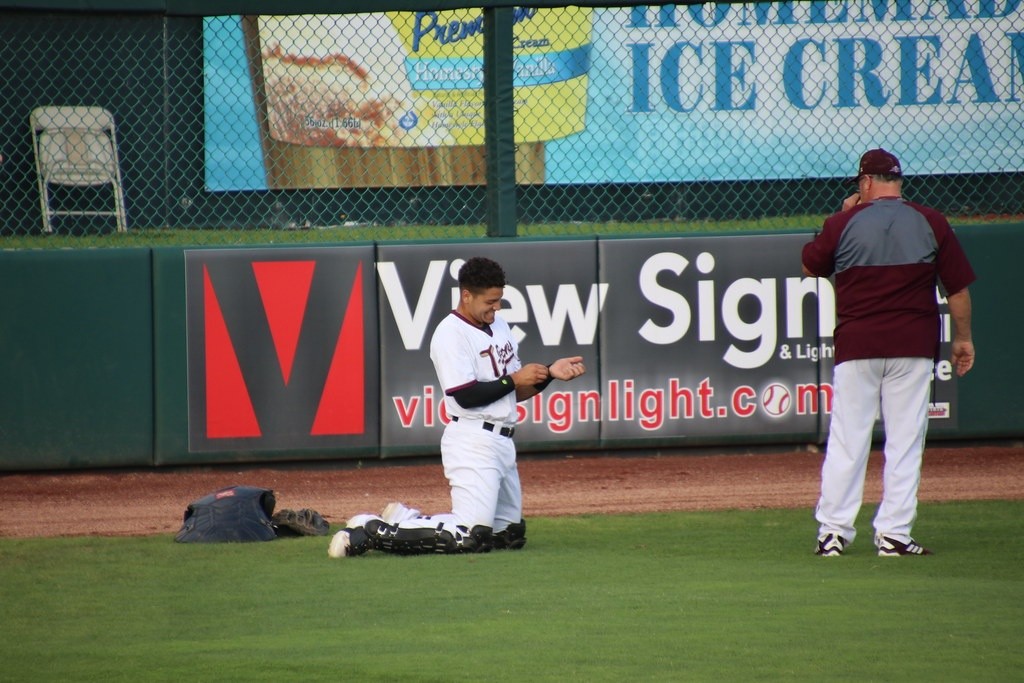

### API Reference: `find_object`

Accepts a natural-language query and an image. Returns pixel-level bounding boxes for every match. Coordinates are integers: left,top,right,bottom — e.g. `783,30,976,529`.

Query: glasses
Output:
854,176,873,192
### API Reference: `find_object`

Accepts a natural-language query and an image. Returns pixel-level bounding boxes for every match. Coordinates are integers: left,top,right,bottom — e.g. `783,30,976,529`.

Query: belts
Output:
452,416,515,439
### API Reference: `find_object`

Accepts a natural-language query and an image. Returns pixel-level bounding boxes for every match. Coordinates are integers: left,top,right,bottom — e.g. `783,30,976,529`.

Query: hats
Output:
847,148,902,184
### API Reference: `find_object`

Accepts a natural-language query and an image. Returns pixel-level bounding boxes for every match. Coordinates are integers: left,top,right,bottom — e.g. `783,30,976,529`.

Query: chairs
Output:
29,105,126,233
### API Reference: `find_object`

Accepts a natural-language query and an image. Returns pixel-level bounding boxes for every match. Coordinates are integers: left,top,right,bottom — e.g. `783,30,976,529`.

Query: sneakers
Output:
877,532,933,556
381,502,409,527
814,533,845,557
328,514,376,559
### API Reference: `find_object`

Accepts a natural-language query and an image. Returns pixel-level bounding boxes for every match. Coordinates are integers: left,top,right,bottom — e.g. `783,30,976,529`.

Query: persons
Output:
799,149,977,557
329,257,586,561
